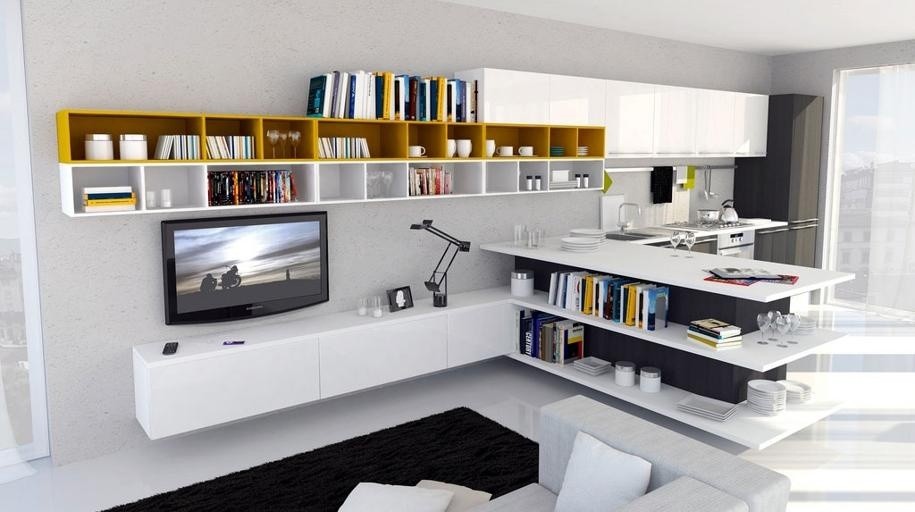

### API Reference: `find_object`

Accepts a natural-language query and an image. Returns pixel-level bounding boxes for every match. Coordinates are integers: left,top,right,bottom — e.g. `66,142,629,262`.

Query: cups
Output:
514,224,545,249
409,139,534,158
356,295,385,318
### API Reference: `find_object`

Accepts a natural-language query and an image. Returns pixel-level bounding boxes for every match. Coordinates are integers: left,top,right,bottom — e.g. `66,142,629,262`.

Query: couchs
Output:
462,395,791,512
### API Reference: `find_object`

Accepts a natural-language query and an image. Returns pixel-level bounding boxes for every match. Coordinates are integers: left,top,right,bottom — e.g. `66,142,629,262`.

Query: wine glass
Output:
671,232,696,258
757,310,801,347
366,172,394,199
266,129,302,159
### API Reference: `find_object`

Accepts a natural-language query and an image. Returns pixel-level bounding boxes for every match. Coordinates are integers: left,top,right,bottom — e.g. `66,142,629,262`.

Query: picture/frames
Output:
386,285,414,313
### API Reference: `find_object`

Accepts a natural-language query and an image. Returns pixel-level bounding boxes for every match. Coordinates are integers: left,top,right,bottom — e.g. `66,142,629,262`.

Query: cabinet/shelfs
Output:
56,109,606,218
480,237,857,462
454,67,769,158
732,94,823,268
133,285,549,442
643,235,717,254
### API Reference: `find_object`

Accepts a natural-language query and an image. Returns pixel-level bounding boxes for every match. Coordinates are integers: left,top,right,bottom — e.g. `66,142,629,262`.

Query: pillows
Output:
338,479,492,511
553,431,652,512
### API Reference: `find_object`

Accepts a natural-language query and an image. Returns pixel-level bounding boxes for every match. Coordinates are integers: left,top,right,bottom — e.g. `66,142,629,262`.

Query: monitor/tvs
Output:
161,210,330,328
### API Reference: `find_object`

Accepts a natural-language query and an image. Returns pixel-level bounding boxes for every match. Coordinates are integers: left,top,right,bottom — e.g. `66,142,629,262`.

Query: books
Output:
687,318,743,350
409,165,453,196
520,271,669,368
306,70,477,124
208,170,297,206
82,185,137,213
713,268,782,280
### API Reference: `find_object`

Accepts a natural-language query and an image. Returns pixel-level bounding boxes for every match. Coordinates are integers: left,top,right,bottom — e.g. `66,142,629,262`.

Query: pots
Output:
697,209,720,224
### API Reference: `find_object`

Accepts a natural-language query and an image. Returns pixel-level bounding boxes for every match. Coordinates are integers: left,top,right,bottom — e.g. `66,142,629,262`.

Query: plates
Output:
550,147,564,155
577,147,588,156
560,228,609,252
794,316,816,335
674,379,812,423
549,181,578,189
574,356,611,377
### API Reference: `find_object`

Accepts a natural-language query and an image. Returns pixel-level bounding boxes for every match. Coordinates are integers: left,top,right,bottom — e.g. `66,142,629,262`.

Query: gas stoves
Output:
666,221,754,232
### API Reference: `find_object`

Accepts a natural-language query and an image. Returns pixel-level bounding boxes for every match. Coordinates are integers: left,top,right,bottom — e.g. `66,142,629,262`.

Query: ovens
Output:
718,230,754,260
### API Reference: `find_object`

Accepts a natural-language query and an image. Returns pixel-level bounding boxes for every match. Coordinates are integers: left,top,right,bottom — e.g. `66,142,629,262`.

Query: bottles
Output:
575,173,589,188
526,175,541,191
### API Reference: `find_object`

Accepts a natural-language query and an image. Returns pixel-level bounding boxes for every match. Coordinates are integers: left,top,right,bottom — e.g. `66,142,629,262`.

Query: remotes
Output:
163,341,178,355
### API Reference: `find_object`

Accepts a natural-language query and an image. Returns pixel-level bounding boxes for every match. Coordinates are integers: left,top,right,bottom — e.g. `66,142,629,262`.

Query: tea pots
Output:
720,199,739,223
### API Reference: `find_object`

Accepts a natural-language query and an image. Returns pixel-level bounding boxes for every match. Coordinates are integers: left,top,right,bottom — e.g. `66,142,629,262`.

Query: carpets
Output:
98,407,539,512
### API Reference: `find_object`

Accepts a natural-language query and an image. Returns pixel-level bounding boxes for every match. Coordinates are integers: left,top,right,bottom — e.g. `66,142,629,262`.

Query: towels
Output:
651,165,696,204
